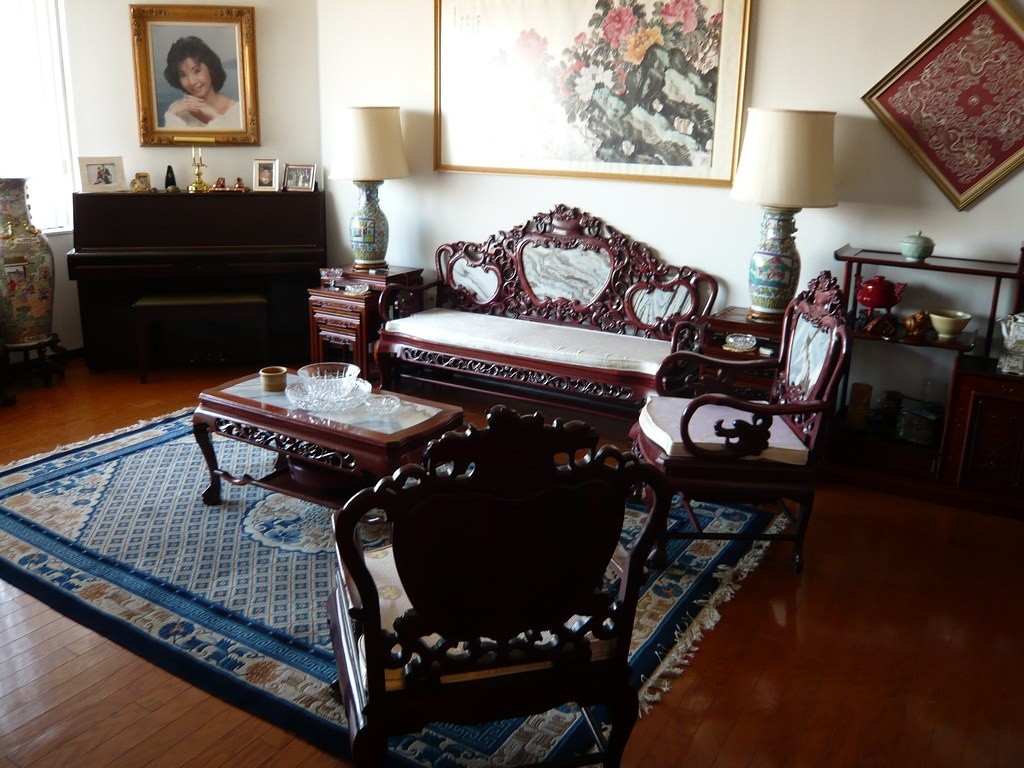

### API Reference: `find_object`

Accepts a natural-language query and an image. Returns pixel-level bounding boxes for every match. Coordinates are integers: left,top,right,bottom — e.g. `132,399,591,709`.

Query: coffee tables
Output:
193,368,465,526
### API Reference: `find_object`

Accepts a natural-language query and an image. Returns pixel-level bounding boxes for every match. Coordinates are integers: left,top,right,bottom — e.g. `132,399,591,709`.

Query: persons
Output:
163,35,241,130
260,166,272,186
95,164,113,185
288,170,310,188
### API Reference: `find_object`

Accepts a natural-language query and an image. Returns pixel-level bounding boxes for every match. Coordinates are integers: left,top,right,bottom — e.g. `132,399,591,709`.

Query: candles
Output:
199,147,202,163
192,145,195,162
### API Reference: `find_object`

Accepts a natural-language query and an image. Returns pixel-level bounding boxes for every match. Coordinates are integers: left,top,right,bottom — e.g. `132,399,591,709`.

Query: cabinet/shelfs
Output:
834,243,1024,519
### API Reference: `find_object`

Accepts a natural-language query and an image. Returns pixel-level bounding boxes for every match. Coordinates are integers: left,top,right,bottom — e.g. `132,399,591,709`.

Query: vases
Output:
0,177,55,344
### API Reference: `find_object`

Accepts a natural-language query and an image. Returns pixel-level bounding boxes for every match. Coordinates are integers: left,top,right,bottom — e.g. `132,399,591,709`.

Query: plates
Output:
285,377,371,412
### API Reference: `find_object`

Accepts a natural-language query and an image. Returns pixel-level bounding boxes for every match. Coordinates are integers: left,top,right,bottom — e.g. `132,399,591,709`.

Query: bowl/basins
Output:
726,334,757,350
364,395,401,416
928,309,972,338
297,362,361,402
901,231,935,262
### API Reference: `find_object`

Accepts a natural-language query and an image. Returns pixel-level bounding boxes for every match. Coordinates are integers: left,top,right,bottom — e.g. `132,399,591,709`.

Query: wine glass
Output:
319,268,343,292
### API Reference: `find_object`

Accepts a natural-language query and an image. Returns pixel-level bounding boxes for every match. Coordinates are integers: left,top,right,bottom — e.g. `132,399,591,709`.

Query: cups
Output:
345,282,369,295
259,366,287,392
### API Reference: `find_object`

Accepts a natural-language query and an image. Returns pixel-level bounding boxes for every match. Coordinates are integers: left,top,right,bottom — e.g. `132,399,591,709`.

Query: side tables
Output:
307,262,424,381
699,307,784,400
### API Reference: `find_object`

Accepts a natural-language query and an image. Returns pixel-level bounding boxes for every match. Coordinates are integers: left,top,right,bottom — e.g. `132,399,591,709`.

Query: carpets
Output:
0,405,790,768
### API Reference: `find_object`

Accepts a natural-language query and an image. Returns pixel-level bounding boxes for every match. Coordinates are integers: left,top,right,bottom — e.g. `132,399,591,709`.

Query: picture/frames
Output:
136,173,150,191
283,162,316,192
861,0,1024,211
254,159,279,192
79,156,126,193
130,4,260,146
433,0,753,187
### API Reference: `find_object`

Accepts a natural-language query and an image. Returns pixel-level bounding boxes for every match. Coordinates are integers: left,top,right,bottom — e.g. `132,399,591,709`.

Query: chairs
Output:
326,403,672,768
629,271,852,573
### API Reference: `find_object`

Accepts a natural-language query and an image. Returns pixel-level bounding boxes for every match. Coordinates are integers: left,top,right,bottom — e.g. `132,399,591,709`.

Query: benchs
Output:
131,296,271,383
373,204,718,423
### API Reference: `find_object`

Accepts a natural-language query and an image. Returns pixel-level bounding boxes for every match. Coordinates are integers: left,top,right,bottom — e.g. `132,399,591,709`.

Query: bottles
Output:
0,178,55,346
846,383,873,431
884,391,899,424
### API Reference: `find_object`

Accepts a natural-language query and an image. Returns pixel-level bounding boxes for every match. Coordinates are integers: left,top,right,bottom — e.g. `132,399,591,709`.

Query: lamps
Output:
327,107,411,272
727,107,841,324
